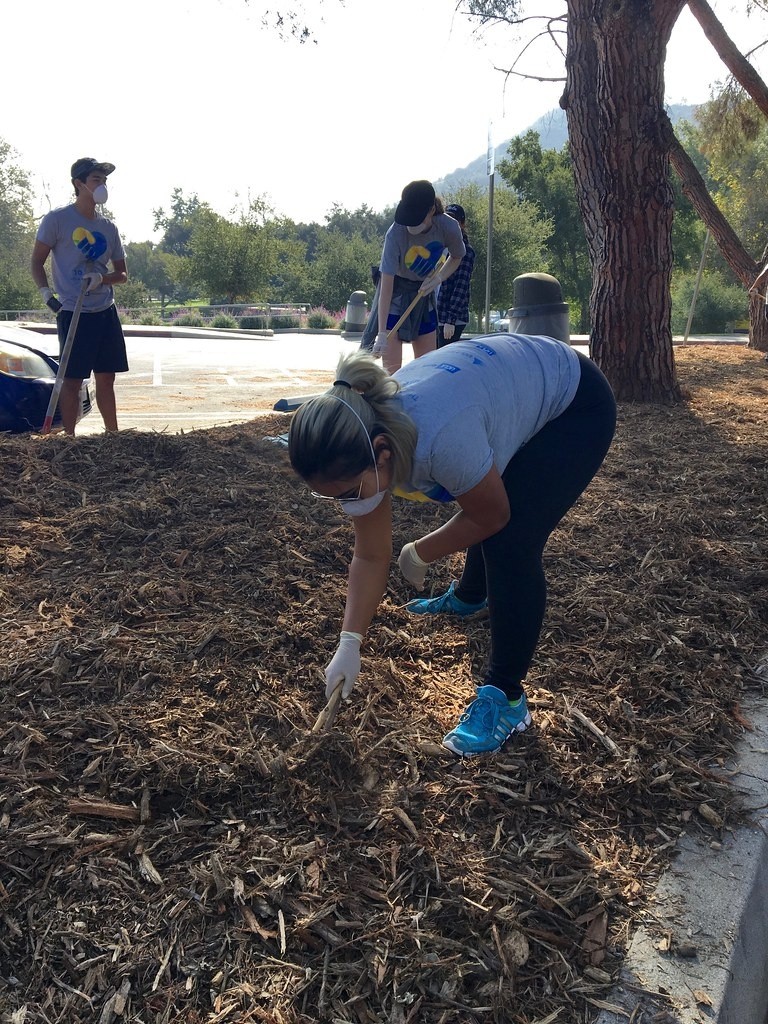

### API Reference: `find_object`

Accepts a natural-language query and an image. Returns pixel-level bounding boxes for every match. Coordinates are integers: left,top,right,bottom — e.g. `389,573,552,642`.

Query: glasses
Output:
311,474,364,501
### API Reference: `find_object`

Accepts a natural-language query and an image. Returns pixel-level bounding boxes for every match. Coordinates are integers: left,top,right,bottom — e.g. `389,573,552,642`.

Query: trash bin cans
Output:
344,289,367,331
506,272,571,346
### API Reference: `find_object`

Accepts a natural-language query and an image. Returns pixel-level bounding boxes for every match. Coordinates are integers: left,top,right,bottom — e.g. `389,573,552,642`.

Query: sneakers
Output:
405,580,487,618
441,685,532,756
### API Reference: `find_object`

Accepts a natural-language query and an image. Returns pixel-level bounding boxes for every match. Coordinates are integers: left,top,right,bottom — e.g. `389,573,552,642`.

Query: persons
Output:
371,180,466,375
30,156,129,435
435,201,475,350
286,330,618,756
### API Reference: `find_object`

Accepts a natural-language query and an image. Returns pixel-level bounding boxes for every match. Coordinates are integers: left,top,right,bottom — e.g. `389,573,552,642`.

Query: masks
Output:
406,205,435,235
338,449,388,517
80,181,108,204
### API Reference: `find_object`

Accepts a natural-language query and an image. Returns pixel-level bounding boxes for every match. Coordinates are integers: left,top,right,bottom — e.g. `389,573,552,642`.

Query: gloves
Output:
398,541,430,591
40,288,61,312
325,631,364,699
82,272,103,293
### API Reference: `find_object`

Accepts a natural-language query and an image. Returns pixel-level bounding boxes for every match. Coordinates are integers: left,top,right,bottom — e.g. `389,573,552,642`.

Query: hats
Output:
394,180,435,227
71,158,116,178
445,204,466,222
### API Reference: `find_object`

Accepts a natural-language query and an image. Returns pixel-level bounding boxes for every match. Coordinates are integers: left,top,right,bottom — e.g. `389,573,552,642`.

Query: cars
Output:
0,323,97,435
